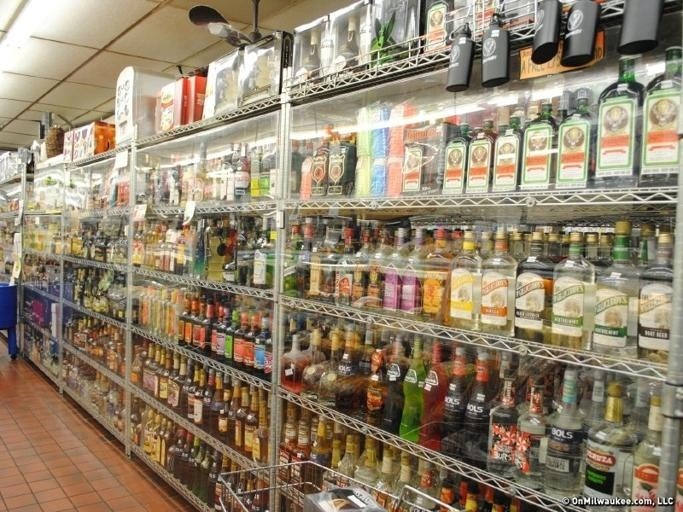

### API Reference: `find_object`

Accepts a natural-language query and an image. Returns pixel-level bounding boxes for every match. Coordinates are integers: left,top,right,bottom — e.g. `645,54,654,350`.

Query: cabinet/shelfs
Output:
0,0,683,512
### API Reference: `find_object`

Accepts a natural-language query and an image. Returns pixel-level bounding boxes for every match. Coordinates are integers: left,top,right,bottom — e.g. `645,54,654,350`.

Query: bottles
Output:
61,0,683,512
